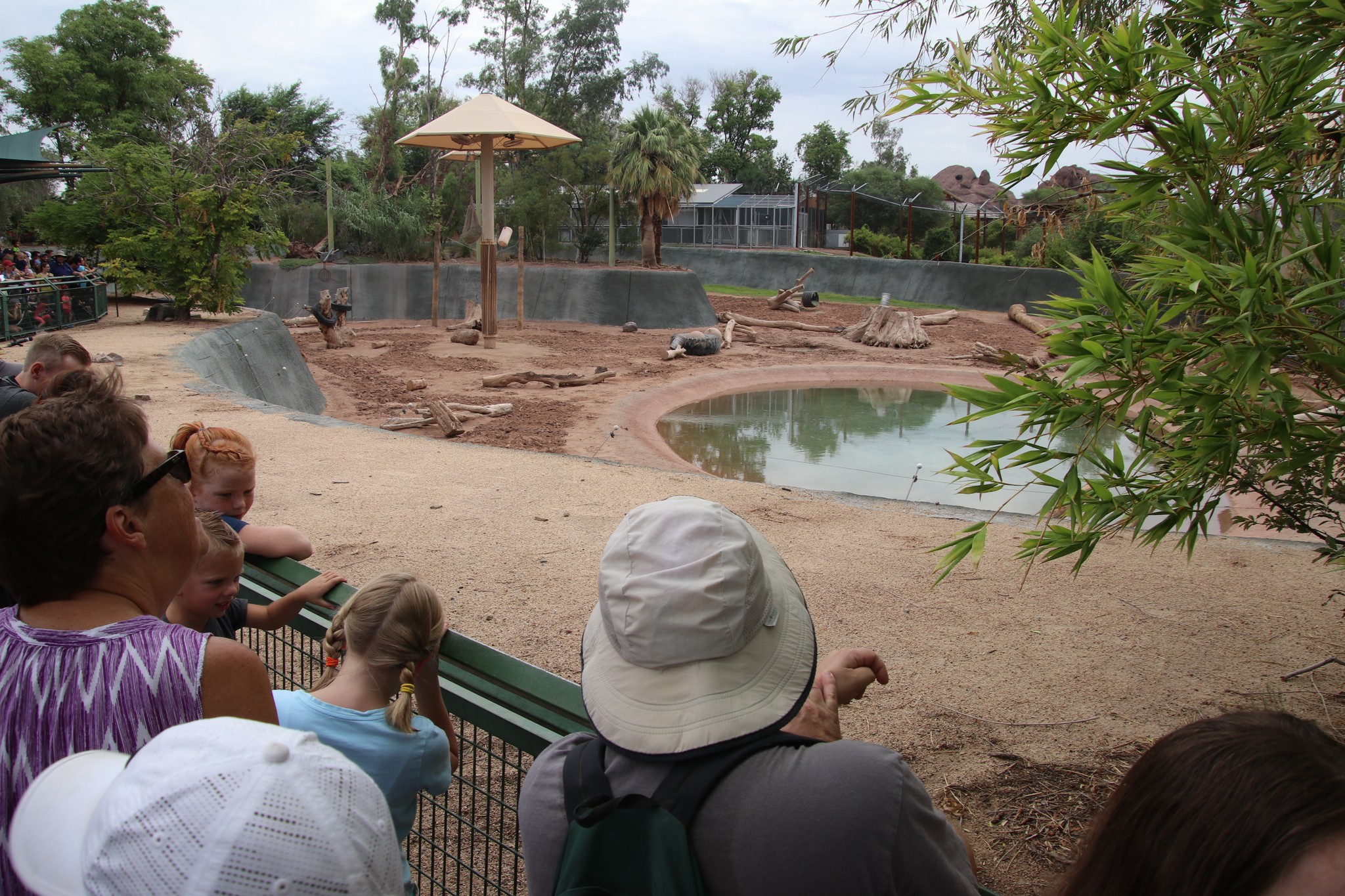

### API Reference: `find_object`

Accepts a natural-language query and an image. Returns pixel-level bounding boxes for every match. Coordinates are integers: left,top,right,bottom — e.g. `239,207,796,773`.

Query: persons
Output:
518,495,980,896
1052,710,1344,896
0,239,108,341
0,331,459,896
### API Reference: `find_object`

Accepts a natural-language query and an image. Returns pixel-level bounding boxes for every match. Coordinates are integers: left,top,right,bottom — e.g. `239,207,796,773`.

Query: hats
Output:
8,299,22,307
3,248,16,254
25,251,32,260
5,716,404,896
3,260,15,266
39,253,48,257
580,494,818,759
52,251,67,256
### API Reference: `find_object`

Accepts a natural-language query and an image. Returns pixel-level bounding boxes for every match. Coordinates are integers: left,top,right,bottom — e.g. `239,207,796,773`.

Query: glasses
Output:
57,256,64,258
74,263,80,267
44,266,50,269
120,449,193,504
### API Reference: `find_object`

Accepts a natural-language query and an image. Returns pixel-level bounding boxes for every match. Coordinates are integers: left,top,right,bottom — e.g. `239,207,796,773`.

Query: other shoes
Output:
64,325,75,328
49,328,56,331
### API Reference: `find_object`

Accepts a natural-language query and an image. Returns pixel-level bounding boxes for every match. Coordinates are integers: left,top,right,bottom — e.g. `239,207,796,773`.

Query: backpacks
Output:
552,721,824,896
